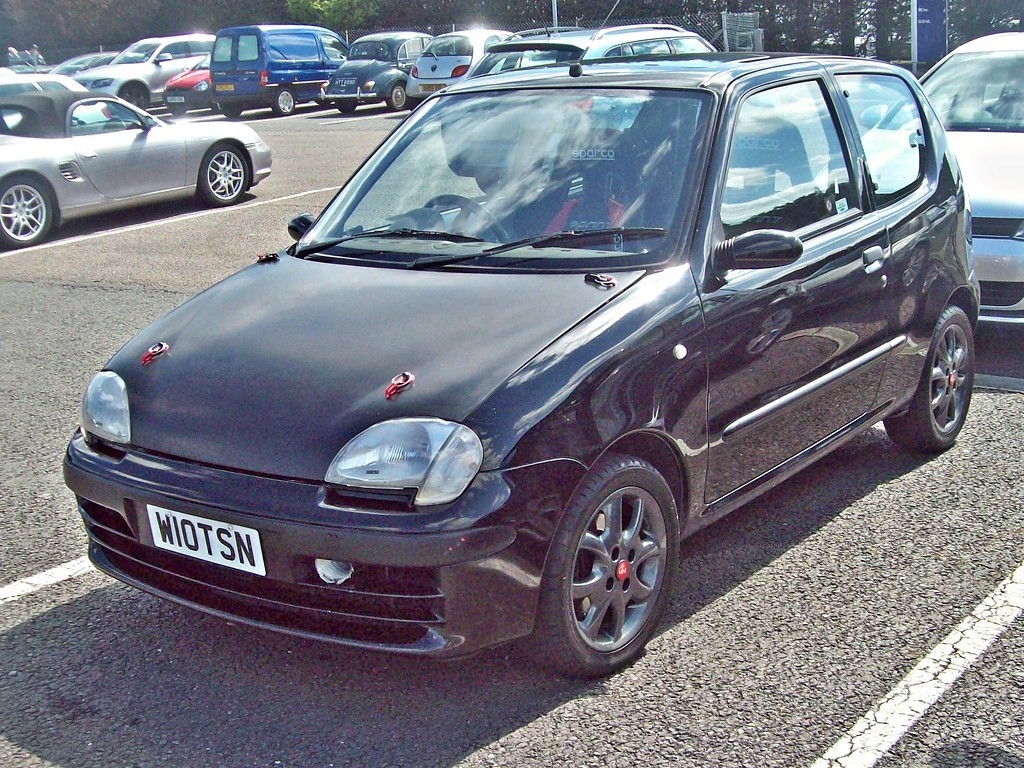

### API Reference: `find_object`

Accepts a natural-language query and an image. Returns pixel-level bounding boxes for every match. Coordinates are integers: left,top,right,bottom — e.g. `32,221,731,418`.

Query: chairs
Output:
693,116,813,204
986,63,1024,120
518,128,643,241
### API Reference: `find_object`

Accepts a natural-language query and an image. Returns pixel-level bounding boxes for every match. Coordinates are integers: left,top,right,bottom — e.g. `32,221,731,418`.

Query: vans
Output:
209,26,357,121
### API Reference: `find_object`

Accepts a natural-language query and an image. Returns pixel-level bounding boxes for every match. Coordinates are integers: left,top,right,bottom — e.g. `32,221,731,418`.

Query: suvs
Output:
462,23,723,135
68,32,215,115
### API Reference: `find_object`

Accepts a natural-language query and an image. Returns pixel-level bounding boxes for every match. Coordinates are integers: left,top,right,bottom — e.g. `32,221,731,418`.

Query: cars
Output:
0,67,113,138
0,88,273,249
812,32,1024,332
46,51,145,99
63,46,982,685
321,29,438,111
162,53,222,117
405,28,525,116
